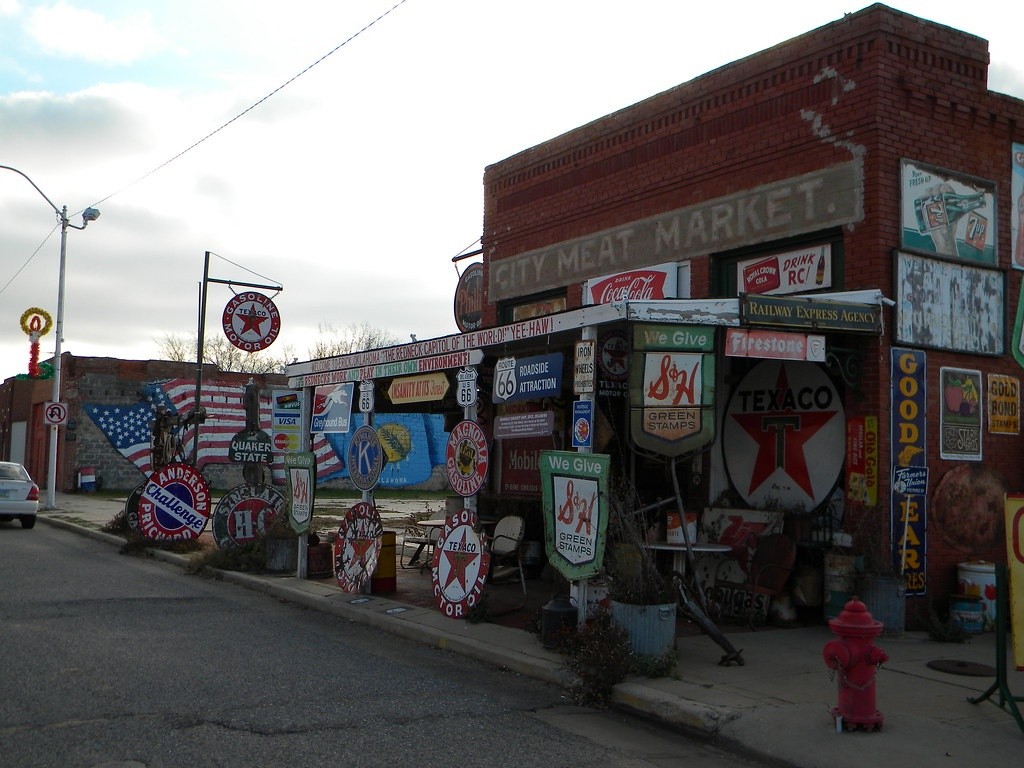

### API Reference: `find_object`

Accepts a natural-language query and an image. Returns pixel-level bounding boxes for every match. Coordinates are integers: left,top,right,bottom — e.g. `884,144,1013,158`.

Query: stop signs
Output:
275,433,290,449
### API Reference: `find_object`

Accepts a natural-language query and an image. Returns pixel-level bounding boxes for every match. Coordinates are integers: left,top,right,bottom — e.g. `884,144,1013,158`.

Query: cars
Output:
0,462,39,528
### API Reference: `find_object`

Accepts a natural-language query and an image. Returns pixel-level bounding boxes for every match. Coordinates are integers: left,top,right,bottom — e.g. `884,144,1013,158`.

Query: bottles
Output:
824,533,856,626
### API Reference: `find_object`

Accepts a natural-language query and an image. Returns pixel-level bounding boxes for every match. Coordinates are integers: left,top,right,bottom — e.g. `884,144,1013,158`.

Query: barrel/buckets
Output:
371,531,397,594
666,511,698,546
958,559,997,628
307,543,334,578
570,574,612,640
78,467,96,493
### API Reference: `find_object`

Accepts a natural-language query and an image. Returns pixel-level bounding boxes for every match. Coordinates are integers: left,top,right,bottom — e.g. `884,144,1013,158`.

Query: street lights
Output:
0,165,102,508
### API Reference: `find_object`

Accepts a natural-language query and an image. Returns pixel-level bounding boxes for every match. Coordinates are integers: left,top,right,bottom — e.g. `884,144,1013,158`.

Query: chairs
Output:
740,534,796,632
482,515,527,615
400,525,443,575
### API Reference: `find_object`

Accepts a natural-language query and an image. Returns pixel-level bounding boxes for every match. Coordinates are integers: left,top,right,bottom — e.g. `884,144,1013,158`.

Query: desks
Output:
642,540,733,574
417,520,446,569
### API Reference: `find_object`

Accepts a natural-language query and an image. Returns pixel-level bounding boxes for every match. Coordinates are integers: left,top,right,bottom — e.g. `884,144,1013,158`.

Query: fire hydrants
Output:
821,595,889,733
